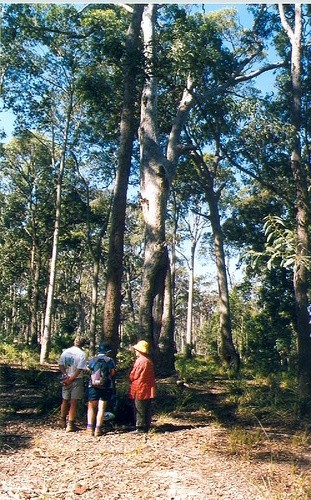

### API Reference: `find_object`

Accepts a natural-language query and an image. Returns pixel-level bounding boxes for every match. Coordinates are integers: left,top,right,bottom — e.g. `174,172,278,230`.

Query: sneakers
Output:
57,419,65,426
94,428,103,435
85,428,94,435
66,423,74,431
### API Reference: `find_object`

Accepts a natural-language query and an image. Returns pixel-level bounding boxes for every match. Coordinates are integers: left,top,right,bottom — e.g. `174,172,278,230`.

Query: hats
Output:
132,340,151,354
97,343,111,353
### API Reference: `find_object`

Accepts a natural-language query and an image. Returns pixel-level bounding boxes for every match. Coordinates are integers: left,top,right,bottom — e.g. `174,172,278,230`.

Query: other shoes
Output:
129,429,148,433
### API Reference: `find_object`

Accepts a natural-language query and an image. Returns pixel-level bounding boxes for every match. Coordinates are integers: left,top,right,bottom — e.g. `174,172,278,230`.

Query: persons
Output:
130,340,157,432
54,336,86,432
87,342,117,437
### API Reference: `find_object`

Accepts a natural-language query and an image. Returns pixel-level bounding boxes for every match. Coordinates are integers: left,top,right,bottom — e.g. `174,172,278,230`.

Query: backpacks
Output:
90,357,111,388
